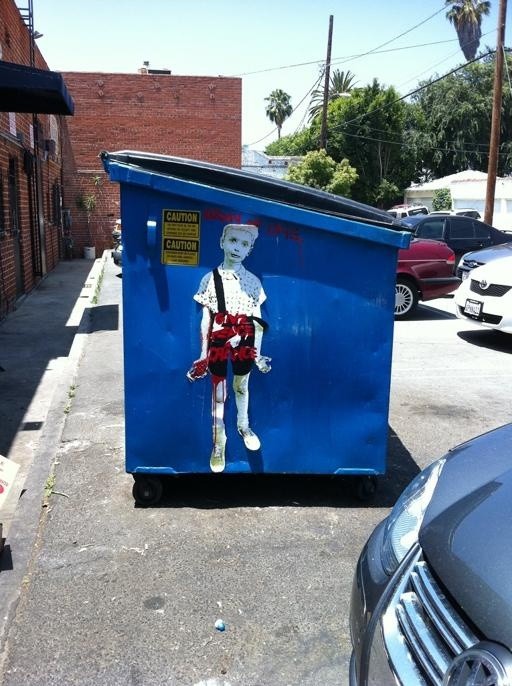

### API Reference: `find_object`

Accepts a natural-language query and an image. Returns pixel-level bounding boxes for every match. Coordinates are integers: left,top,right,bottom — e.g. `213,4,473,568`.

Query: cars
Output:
386,204,512,333
348,422,512,686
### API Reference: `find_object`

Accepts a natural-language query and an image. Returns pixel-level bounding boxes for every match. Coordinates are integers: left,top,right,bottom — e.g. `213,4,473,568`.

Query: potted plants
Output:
76,193,97,260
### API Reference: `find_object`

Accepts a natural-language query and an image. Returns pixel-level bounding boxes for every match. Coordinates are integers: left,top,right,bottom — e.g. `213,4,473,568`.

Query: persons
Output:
185,223,273,474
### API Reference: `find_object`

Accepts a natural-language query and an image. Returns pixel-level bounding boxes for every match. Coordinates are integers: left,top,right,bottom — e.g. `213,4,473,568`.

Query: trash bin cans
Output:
100,148,414,507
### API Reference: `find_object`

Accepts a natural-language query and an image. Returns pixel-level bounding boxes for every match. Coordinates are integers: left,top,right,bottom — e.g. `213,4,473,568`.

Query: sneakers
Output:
237,426,261,451
210,439,227,473
